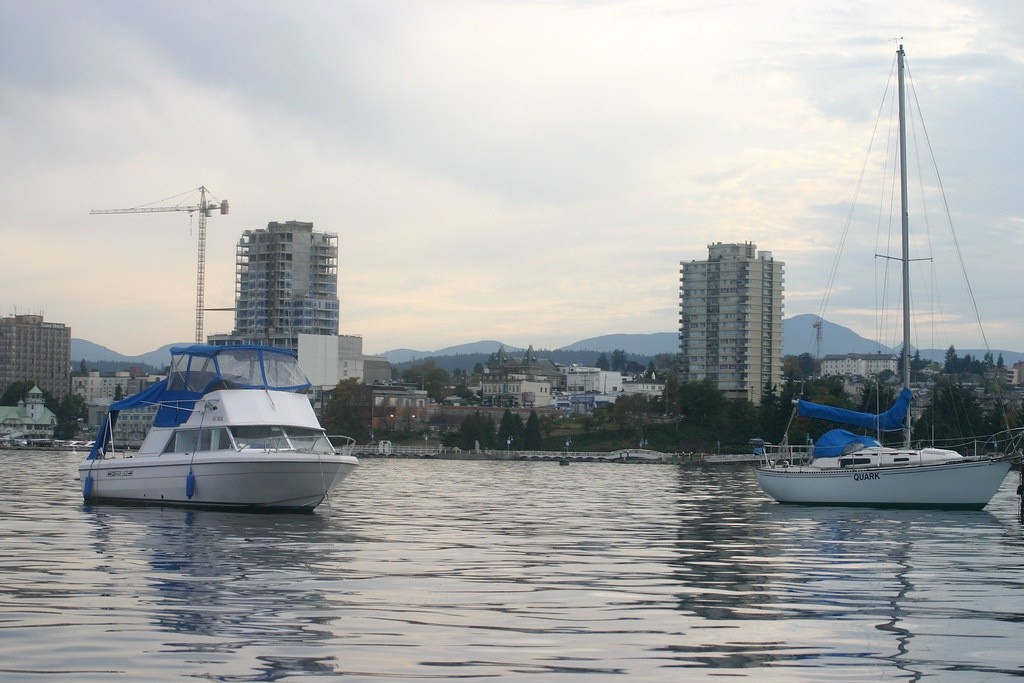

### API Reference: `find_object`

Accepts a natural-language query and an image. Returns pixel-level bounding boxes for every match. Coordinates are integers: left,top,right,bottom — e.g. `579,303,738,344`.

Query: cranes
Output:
90,184,230,341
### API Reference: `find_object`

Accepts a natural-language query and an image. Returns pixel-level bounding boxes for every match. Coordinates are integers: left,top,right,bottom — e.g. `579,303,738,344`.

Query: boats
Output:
77,342,361,516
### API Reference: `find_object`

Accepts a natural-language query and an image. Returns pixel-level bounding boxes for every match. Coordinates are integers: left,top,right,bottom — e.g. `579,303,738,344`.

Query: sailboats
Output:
750,44,1022,511
